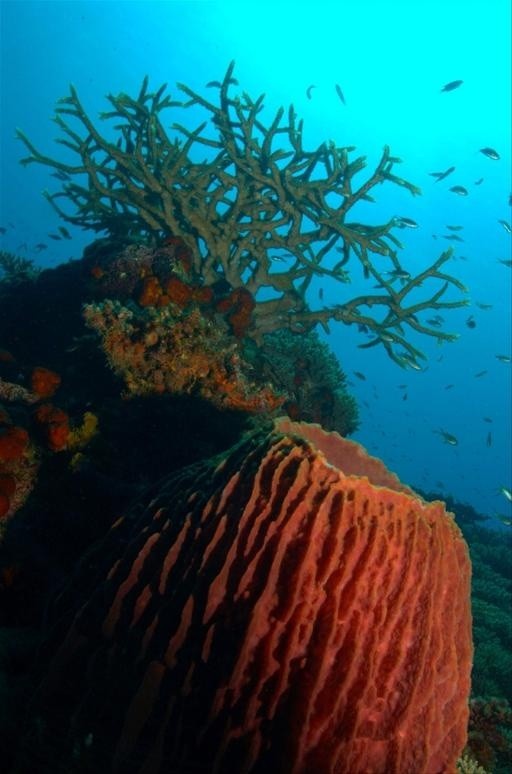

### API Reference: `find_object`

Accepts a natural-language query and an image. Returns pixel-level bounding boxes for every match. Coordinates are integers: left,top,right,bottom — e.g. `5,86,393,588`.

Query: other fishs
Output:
318,146,511,448
